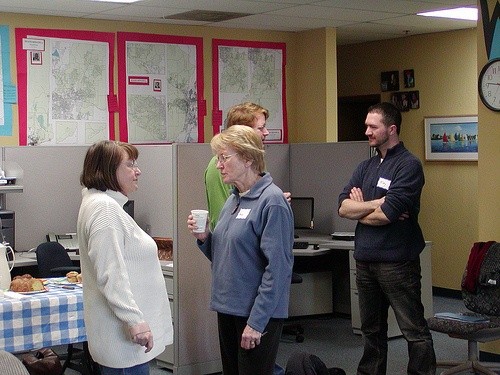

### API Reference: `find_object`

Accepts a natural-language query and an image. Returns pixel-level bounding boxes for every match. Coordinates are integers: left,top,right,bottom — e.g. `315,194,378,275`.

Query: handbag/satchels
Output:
20,347,62,375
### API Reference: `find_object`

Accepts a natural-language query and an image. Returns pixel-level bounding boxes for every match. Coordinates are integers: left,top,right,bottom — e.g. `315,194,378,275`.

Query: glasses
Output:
255,124,267,131
125,160,141,169
216,153,239,163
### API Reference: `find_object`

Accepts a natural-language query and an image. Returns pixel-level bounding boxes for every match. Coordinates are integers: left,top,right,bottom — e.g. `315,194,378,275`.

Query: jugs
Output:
0,243,14,290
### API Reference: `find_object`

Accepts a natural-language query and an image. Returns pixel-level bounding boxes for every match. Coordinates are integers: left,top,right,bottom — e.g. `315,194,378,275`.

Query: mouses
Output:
314,244,320,250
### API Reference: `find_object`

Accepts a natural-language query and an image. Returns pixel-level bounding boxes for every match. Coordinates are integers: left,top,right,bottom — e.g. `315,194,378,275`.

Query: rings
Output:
251,342,255,344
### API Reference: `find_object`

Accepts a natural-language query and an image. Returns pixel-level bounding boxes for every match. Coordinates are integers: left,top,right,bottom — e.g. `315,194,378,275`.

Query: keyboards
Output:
293,242,308,249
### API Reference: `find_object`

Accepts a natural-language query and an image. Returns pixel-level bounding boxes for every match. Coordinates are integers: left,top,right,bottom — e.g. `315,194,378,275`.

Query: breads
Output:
66,271,82,283
10,274,49,291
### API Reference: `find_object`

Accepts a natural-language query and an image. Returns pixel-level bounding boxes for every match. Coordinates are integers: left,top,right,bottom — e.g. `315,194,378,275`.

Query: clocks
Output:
477,57,500,112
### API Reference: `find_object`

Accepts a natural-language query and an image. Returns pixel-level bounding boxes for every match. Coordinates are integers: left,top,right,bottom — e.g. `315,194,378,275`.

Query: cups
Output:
191,209,208,233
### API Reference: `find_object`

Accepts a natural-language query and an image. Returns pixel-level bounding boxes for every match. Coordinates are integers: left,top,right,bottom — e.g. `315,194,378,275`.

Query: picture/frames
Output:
423,116,479,162
380,69,420,112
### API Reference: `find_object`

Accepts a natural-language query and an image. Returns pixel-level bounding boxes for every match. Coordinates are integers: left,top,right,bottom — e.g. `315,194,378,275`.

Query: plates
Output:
50,280,80,284
5,289,47,294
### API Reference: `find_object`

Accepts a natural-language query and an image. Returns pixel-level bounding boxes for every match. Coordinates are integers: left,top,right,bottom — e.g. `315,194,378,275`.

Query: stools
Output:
279,273,305,343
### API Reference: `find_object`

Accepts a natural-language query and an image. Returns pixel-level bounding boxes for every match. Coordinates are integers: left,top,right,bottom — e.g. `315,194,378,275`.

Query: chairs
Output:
35,240,81,277
426,240,500,375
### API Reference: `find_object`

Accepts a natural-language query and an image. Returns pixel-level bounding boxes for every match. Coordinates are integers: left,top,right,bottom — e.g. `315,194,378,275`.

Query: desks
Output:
0,234,434,375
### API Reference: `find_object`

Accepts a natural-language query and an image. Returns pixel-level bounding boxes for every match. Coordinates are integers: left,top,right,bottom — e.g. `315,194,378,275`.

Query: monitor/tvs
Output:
289,197,314,238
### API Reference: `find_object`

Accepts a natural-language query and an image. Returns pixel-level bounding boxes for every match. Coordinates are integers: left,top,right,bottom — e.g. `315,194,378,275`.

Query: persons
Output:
77,140,174,375
187,125,294,375
204,102,292,232
338,103,436,375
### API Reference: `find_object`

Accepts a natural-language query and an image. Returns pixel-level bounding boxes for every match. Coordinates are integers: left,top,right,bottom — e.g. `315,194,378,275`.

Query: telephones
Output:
45,232,80,251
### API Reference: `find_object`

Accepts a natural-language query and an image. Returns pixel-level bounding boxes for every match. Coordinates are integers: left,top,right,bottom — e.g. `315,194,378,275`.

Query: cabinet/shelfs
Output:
154,271,174,370
